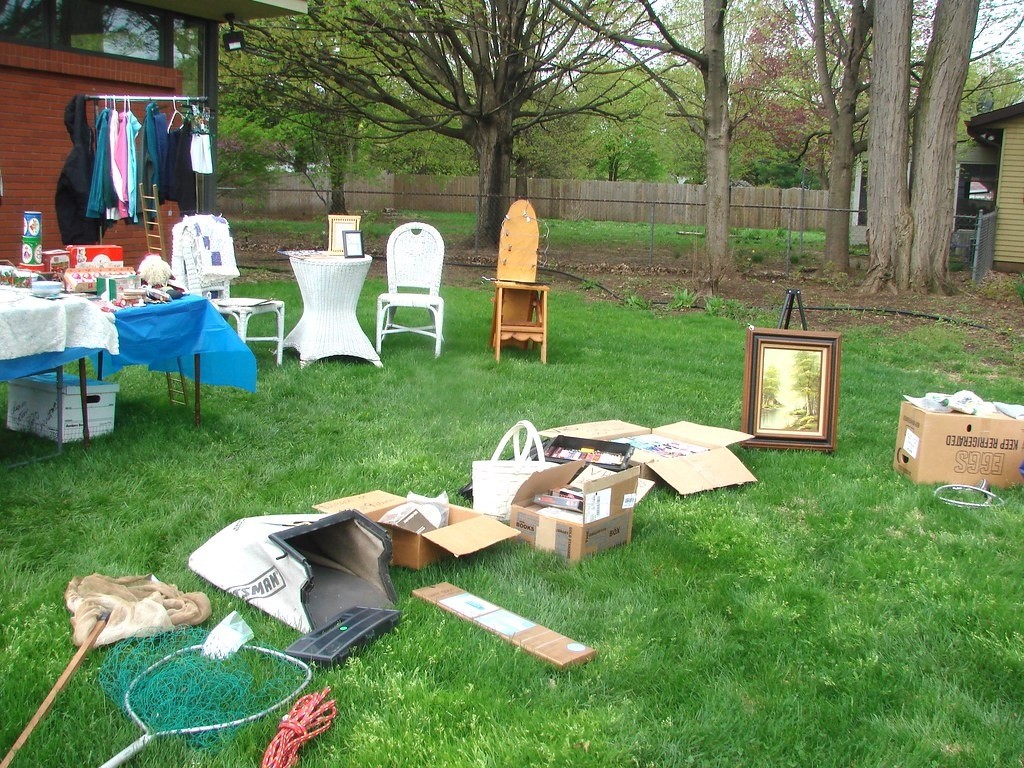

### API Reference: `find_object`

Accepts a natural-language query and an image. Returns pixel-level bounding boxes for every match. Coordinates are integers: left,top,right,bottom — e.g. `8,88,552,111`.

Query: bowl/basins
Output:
31,281,62,294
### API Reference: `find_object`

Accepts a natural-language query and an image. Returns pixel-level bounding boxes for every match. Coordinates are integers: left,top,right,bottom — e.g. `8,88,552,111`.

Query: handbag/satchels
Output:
472,420,558,520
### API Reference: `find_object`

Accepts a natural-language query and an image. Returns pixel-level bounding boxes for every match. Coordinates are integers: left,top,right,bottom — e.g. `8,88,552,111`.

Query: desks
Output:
271,254,384,370
0,276,257,476
492,281,551,365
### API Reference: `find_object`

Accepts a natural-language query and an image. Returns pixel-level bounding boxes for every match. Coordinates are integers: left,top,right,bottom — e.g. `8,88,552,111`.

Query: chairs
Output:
376,222,446,358
172,213,285,368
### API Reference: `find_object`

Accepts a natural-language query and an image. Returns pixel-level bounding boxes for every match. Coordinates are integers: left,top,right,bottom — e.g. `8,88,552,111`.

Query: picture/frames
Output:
342,229,364,258
328,214,361,255
740,325,841,452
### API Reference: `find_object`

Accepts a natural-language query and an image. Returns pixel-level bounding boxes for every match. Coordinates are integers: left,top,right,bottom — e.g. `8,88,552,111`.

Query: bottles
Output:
23,211,42,238
22,236,43,264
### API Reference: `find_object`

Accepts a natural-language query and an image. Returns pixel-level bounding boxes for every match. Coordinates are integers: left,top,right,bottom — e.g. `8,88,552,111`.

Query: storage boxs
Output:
43,243,135,294
8,370,120,443
313,419,757,669
893,402,1024,489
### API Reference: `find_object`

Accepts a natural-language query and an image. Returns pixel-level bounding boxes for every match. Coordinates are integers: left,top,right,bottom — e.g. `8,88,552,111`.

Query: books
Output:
533,485,583,513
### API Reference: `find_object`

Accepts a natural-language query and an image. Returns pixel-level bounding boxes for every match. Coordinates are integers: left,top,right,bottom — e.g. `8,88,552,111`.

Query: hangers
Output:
167,97,216,136
104,95,131,113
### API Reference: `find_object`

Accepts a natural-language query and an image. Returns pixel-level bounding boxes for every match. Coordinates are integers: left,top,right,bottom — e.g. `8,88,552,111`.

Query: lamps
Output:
223,12,244,53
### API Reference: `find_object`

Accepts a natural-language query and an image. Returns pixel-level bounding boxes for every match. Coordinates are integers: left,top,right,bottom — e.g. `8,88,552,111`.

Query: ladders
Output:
139,183,167,261
